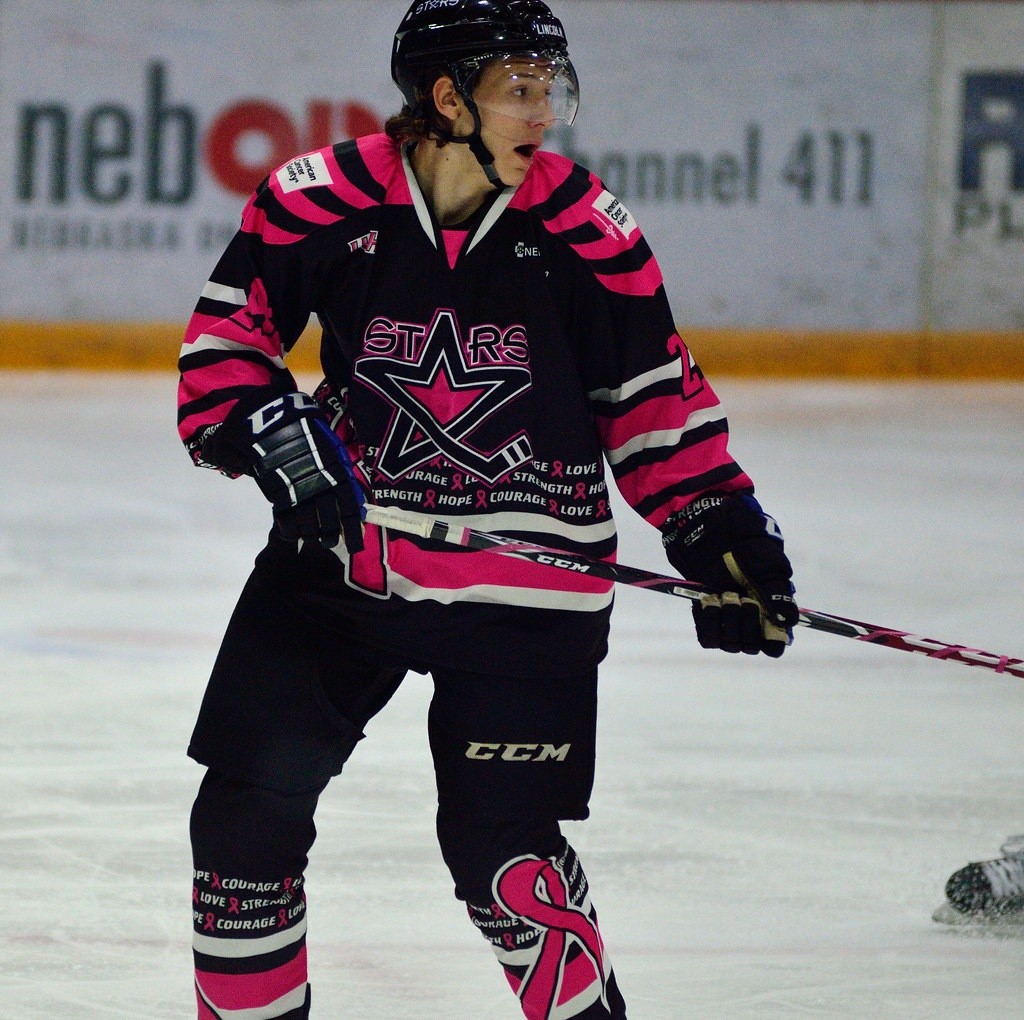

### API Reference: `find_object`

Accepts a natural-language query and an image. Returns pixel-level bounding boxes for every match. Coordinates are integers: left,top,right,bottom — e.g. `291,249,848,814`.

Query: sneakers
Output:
944,830,1024,925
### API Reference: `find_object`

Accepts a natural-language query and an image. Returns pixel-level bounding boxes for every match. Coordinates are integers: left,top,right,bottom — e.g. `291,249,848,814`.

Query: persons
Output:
930,834,1024,932
172,1,804,1020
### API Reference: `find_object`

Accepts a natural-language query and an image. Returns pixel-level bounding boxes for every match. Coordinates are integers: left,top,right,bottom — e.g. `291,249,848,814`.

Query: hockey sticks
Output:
362,502,1024,677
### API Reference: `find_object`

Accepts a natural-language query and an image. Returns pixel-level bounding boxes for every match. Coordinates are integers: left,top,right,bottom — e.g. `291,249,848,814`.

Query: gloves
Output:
227,390,369,556
661,494,800,659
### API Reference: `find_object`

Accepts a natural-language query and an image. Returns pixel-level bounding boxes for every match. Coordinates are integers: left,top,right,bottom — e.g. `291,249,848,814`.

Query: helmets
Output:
389,0,581,130
